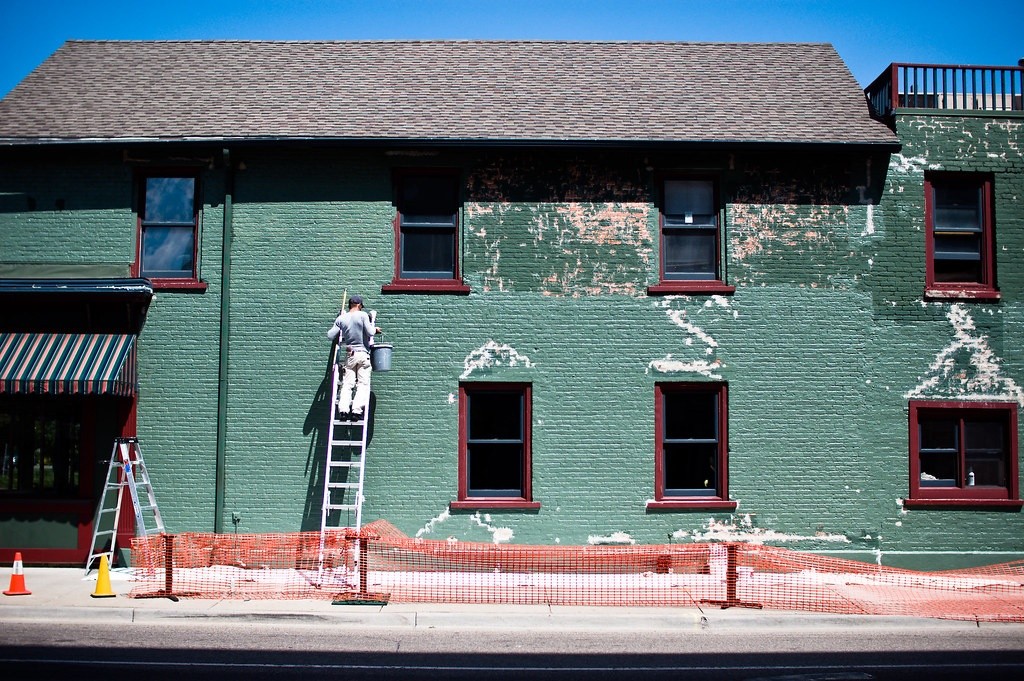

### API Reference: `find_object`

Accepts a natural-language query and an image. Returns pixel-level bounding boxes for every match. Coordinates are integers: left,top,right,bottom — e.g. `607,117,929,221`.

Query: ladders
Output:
84,436,180,580
314,309,377,591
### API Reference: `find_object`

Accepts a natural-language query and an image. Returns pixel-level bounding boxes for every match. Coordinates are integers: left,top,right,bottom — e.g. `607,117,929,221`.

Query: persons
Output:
326,296,381,424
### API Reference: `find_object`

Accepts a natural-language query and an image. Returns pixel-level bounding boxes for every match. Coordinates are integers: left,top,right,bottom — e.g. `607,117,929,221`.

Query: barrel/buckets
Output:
370,344,393,373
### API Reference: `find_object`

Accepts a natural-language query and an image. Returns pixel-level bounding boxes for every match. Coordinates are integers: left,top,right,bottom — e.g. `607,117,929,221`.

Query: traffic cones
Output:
90,554,117,598
2,552,32,596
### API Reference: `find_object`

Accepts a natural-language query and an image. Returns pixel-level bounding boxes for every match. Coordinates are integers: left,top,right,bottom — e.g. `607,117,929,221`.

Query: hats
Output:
348,296,365,308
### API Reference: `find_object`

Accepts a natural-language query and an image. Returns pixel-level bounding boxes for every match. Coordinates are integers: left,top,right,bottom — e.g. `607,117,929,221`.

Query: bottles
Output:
968,468,975,487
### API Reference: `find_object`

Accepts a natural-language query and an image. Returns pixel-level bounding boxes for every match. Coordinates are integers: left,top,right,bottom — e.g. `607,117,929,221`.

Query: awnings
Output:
0,333,139,400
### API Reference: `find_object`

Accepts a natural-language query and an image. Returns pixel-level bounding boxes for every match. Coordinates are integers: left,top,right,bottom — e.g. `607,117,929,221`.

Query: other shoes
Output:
339,412,348,421
350,413,358,422
